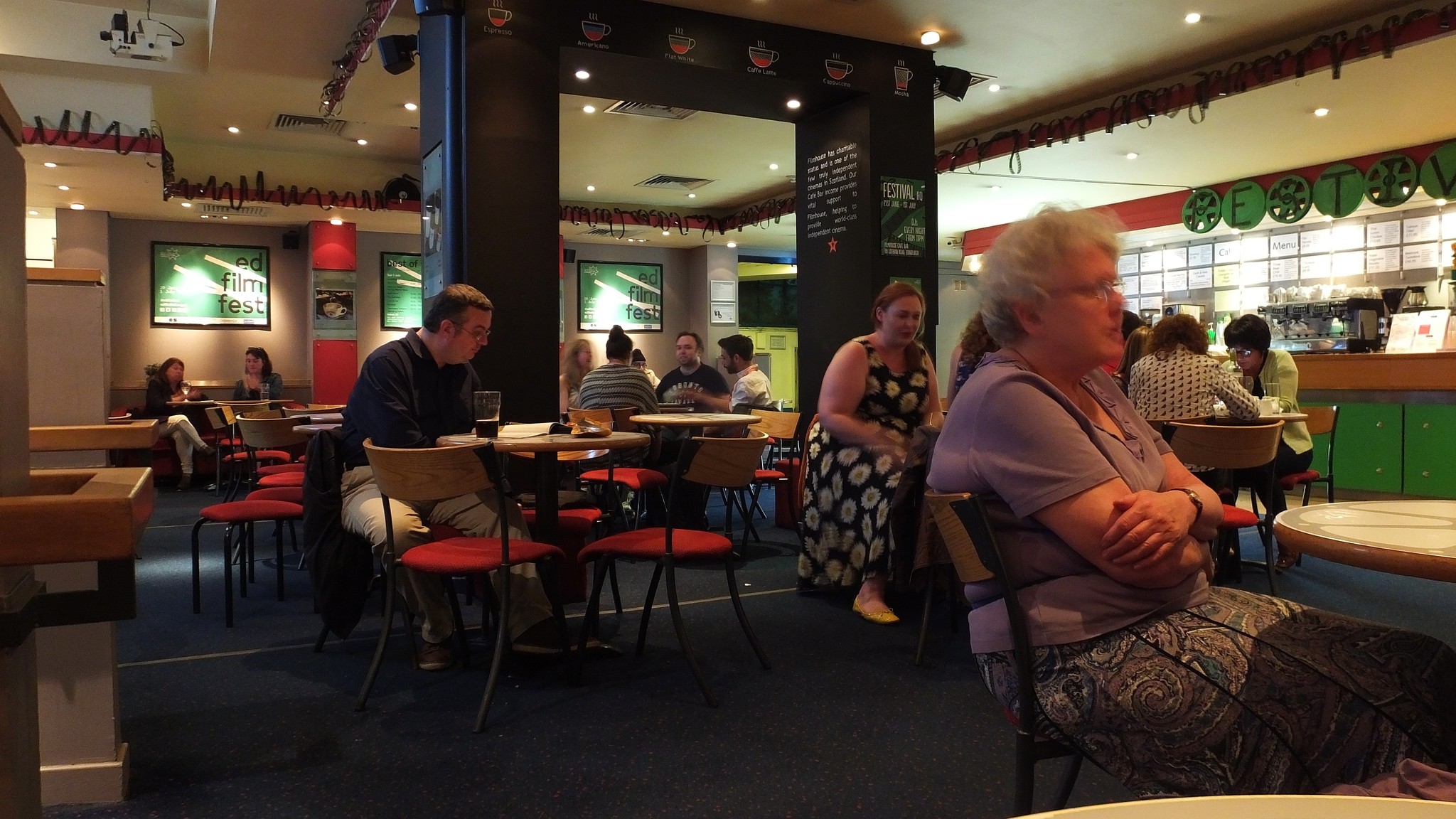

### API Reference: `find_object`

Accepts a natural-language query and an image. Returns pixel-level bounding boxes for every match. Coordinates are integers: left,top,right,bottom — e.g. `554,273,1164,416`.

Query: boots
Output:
176,473,193,489
199,446,217,457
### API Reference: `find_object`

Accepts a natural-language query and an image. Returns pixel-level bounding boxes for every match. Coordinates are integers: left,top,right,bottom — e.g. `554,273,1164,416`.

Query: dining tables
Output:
1272,499,1456,583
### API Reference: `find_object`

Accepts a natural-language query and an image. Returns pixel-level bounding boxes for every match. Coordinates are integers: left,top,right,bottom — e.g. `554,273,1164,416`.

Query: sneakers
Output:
512,626,601,655
418,642,452,670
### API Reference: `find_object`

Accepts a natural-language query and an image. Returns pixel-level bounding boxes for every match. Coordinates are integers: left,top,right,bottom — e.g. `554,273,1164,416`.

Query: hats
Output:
632,349,646,363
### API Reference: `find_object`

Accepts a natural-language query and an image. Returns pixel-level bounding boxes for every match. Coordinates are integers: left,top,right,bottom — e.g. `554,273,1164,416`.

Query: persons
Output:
630,346,664,397
146,357,216,490
338,281,617,670
1127,311,1260,567
653,331,731,529
1214,314,1314,570
932,205,1456,807
576,322,663,525
1111,323,1160,401
677,333,774,531
946,311,1003,413
796,281,945,625
559,339,597,416
231,347,282,474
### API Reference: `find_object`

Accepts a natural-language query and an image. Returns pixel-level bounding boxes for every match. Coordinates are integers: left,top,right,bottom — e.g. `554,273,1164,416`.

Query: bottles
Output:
1226,347,1243,384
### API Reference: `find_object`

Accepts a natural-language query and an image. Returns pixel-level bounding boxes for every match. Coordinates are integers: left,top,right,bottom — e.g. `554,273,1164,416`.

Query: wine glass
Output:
181,381,191,402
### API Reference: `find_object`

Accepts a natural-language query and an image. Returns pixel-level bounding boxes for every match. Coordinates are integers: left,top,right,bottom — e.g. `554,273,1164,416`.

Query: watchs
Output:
1168,486,1206,531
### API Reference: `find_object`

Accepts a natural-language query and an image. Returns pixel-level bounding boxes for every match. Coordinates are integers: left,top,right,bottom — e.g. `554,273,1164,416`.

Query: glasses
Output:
450,320,491,342
248,347,264,354
633,364,647,368
580,351,591,354
1226,348,1256,358
1048,278,1127,304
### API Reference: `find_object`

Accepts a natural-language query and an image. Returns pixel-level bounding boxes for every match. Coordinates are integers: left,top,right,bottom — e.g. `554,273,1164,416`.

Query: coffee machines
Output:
1257,297,1384,353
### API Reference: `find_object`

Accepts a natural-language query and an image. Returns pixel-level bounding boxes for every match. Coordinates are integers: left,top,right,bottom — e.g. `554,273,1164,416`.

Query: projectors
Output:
100,9,172,63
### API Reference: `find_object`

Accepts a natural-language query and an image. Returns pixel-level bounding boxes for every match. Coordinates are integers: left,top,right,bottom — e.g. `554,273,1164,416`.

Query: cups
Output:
1237,376,1254,395
473,391,500,439
260,383,270,401
323,303,347,318
1264,383,1280,414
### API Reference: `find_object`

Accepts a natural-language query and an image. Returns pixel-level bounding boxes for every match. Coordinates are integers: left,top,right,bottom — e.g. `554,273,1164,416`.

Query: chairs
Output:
351,435,583,735
120,345,1344,625
921,487,1083,818
572,427,776,709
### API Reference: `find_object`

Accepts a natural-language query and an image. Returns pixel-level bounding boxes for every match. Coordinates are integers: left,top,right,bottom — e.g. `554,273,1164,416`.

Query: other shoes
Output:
853,594,900,624
1275,539,1300,567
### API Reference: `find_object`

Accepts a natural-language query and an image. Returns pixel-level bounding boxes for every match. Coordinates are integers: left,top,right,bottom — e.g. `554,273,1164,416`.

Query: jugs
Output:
1399,286,1429,306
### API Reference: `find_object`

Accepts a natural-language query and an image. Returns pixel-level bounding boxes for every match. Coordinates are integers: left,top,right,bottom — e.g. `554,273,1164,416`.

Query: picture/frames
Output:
710,280,736,303
576,260,665,334
379,251,424,331
711,302,736,324
148,241,272,328
769,335,786,350
